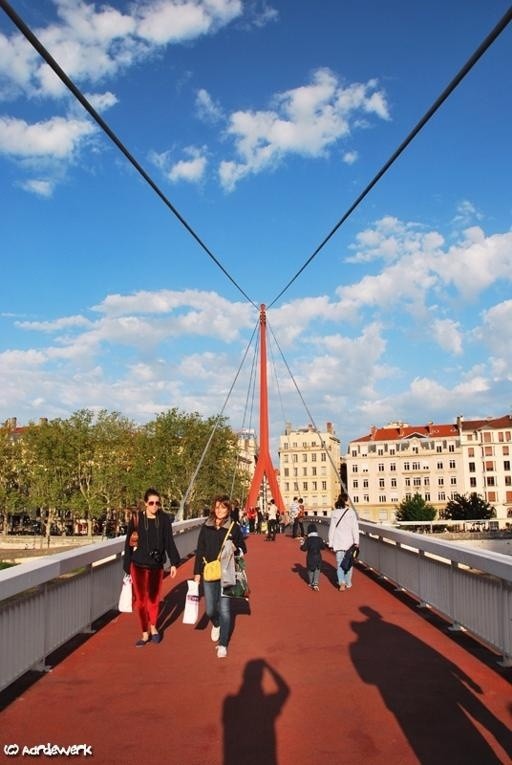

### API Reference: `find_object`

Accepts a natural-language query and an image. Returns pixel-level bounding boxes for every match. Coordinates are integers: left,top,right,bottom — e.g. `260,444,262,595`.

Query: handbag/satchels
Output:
129,510,139,547
220,547,250,599
203,560,221,581
118,572,133,613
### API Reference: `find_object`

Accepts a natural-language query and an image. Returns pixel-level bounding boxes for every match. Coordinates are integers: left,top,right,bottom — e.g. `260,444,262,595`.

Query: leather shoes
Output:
136,636,150,646
150,631,161,643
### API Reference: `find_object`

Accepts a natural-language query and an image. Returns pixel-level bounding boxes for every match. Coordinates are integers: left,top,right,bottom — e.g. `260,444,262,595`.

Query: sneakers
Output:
211,625,220,642
216,645,227,658
308,584,319,592
339,583,352,592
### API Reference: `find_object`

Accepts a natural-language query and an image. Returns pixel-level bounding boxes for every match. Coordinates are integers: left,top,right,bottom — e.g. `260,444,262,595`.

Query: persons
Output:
300,523,325,591
123,488,181,649
230,496,305,541
193,495,247,658
328,493,360,592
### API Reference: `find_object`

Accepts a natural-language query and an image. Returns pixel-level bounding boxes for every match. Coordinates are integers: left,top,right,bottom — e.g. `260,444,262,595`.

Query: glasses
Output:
148,502,160,506
